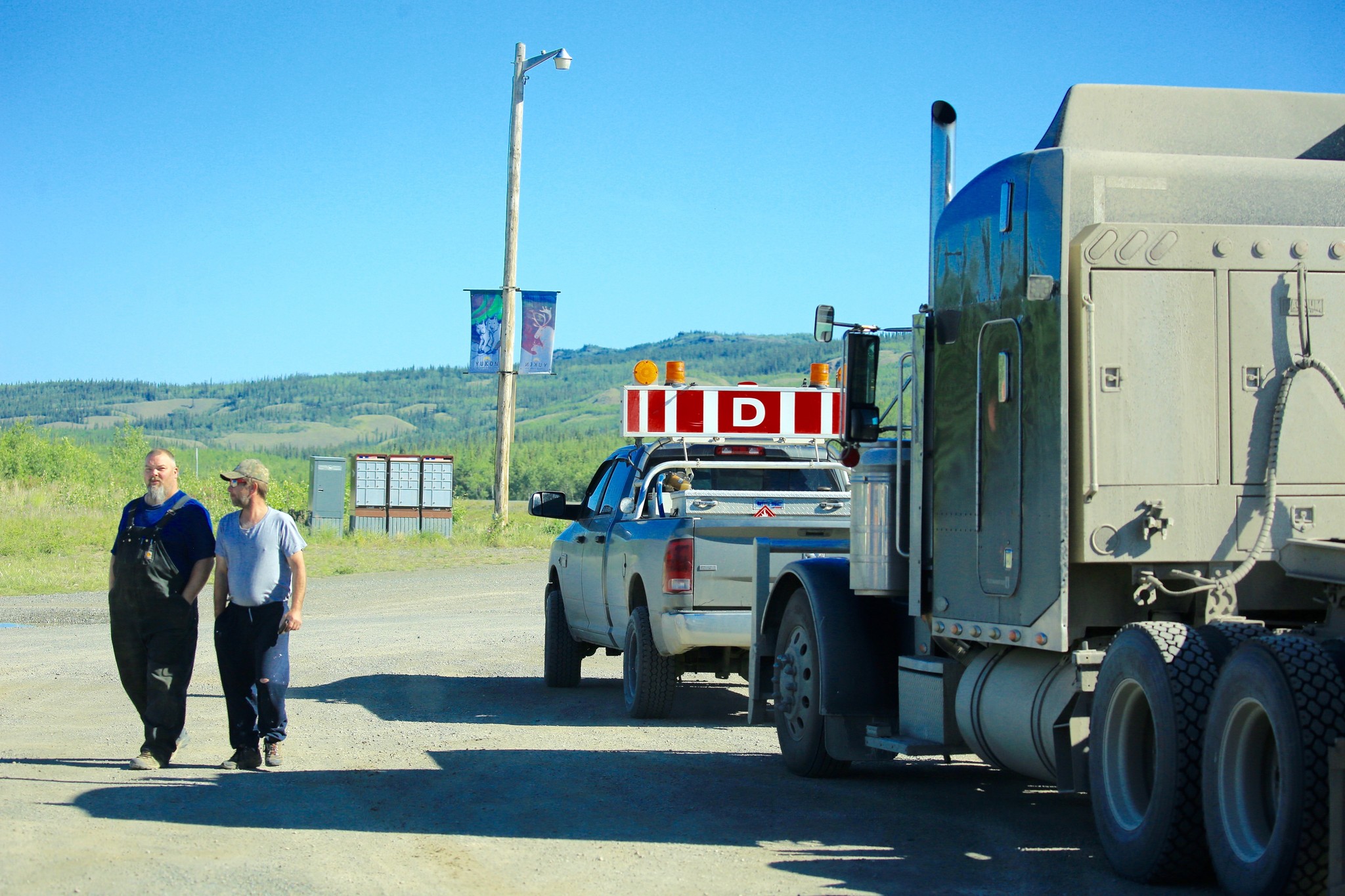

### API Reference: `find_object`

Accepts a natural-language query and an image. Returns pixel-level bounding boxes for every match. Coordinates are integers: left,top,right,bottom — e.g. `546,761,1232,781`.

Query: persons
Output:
108,448,216,769
214,458,307,770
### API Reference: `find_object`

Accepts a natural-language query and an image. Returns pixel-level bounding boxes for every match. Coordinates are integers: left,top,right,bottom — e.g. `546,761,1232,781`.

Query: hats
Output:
220,459,269,485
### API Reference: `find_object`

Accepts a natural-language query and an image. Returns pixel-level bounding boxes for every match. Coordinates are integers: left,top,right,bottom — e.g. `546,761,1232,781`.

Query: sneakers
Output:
221,746,262,769
130,751,167,770
169,728,190,761
263,740,284,766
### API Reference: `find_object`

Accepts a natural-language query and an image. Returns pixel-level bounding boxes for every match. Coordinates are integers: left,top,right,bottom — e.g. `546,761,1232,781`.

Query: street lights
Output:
495,39,573,540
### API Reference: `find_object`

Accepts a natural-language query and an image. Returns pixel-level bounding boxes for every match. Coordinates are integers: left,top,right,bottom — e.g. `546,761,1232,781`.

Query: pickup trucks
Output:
528,363,873,722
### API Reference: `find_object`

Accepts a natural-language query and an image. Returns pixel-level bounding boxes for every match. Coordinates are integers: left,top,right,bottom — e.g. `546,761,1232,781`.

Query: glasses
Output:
230,479,245,488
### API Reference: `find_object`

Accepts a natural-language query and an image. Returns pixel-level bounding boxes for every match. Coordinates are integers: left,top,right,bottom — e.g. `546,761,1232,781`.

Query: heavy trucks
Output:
759,83,1344,896
